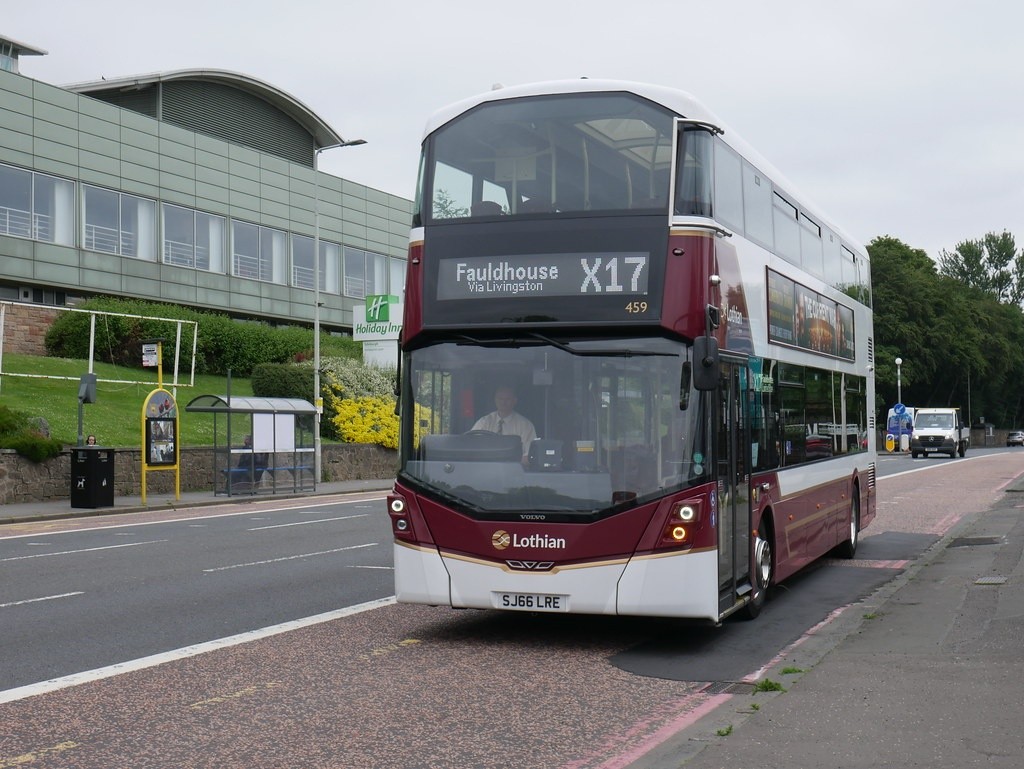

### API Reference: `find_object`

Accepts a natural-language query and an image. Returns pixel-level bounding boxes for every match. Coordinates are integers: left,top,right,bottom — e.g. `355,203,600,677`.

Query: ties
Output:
496,419,505,435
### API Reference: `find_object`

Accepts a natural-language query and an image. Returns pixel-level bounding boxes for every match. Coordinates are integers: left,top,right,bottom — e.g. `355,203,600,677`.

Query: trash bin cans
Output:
70,447,115,508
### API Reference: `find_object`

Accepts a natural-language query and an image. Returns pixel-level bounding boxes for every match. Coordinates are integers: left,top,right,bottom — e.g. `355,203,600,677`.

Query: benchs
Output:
219,466,310,496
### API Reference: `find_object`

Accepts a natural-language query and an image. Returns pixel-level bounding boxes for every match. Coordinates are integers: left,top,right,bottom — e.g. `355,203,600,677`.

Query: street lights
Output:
895,357,902,452
312,138,369,482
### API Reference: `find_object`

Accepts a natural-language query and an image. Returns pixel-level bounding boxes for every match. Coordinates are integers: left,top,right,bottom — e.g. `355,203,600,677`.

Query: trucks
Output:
910,409,970,459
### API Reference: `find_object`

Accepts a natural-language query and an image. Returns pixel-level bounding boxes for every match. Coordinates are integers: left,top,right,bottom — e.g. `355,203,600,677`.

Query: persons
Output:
152,422,174,463
226,434,270,490
86,434,96,445
159,398,170,418
470,385,537,465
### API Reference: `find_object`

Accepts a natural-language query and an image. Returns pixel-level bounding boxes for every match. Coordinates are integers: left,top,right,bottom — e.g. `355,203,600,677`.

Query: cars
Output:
1006,431,1024,447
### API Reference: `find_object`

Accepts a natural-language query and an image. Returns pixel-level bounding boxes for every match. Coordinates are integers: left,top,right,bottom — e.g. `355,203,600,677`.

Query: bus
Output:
384,75,878,628
